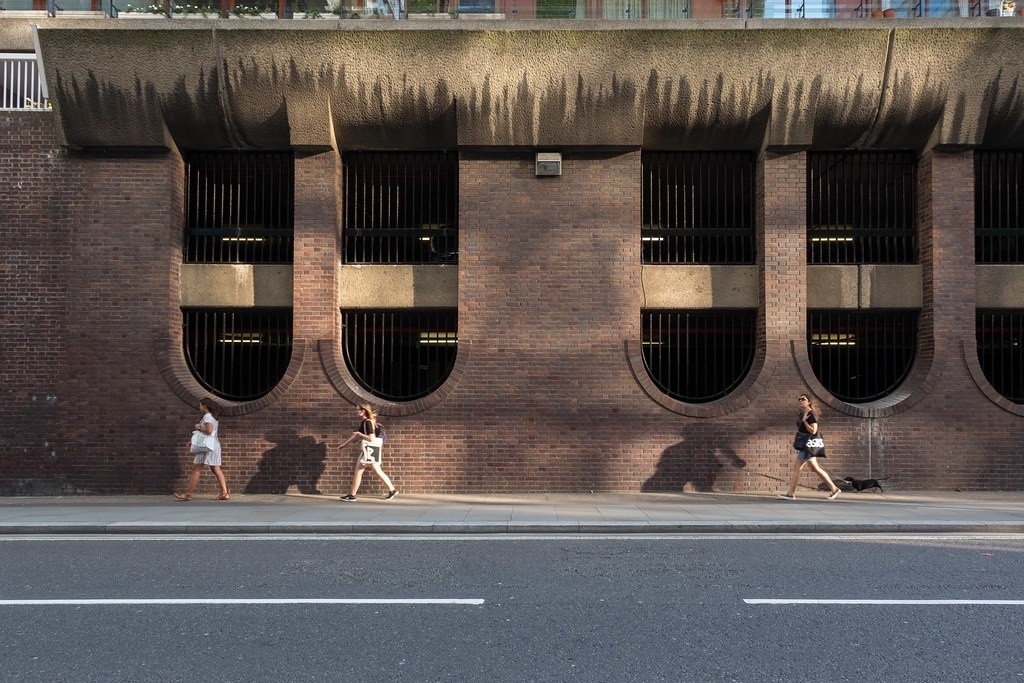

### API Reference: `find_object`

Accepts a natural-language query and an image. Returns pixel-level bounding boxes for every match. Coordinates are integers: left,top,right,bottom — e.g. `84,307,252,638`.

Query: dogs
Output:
844,476,890,493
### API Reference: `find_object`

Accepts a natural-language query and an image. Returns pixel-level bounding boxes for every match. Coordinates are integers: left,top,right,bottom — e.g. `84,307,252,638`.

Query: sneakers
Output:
385,490,399,500
826,489,841,500
339,494,357,502
778,492,795,500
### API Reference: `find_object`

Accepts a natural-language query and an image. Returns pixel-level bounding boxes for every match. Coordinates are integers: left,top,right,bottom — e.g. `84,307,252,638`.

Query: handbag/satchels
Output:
805,433,827,459
190,431,214,453
794,432,810,450
359,438,383,464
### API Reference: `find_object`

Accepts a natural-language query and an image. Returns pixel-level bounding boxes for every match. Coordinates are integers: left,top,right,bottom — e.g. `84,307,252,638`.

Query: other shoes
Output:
174,492,191,501
213,492,229,500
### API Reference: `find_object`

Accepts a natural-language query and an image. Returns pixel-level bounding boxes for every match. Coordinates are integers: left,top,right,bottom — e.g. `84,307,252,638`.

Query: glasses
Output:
799,398,807,401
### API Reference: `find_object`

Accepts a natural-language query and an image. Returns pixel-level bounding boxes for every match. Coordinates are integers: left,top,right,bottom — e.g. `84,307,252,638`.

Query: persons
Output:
174,398,230,500
337,402,399,501
777,394,842,500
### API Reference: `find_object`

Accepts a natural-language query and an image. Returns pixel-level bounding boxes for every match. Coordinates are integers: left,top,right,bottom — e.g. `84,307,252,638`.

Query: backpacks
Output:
375,423,386,444
